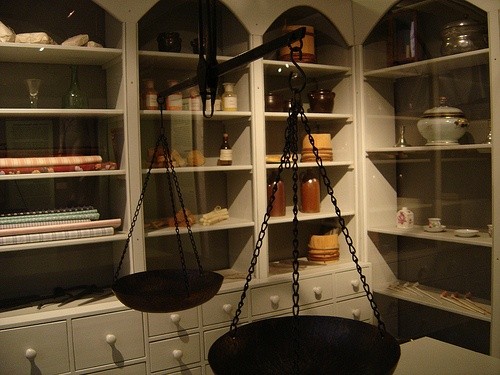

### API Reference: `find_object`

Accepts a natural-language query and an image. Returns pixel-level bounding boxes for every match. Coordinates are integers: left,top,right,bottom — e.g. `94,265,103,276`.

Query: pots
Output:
265,93,281,112
439,18,484,55
307,89,335,113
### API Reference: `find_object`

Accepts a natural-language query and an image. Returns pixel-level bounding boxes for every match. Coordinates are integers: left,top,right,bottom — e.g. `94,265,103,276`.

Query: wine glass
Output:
23,78,42,108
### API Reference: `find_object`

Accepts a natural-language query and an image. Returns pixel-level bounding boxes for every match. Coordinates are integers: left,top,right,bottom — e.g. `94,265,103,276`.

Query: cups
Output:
184,150,205,166
428,217,441,229
396,206,415,229
488,224,493,237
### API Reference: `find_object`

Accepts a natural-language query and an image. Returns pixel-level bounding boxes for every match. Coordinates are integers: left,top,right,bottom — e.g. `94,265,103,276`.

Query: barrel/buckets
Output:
279,24,314,63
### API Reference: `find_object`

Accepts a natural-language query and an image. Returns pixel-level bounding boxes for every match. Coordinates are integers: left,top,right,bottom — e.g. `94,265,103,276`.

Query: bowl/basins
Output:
416,96,470,147
156,31,181,52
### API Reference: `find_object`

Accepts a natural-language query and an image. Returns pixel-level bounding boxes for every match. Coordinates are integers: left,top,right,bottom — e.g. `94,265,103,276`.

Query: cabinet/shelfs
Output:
0,0,500,375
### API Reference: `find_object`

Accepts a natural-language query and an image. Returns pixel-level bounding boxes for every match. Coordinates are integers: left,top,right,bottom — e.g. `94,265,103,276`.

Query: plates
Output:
454,228,479,238
424,225,447,232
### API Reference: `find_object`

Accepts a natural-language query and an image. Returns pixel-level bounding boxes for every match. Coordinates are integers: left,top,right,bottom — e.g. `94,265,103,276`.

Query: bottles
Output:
142,80,158,110
300,169,320,213
219,133,232,166
167,79,183,110
62,63,89,109
267,171,286,217
187,86,201,111
221,82,237,111
151,127,170,168
